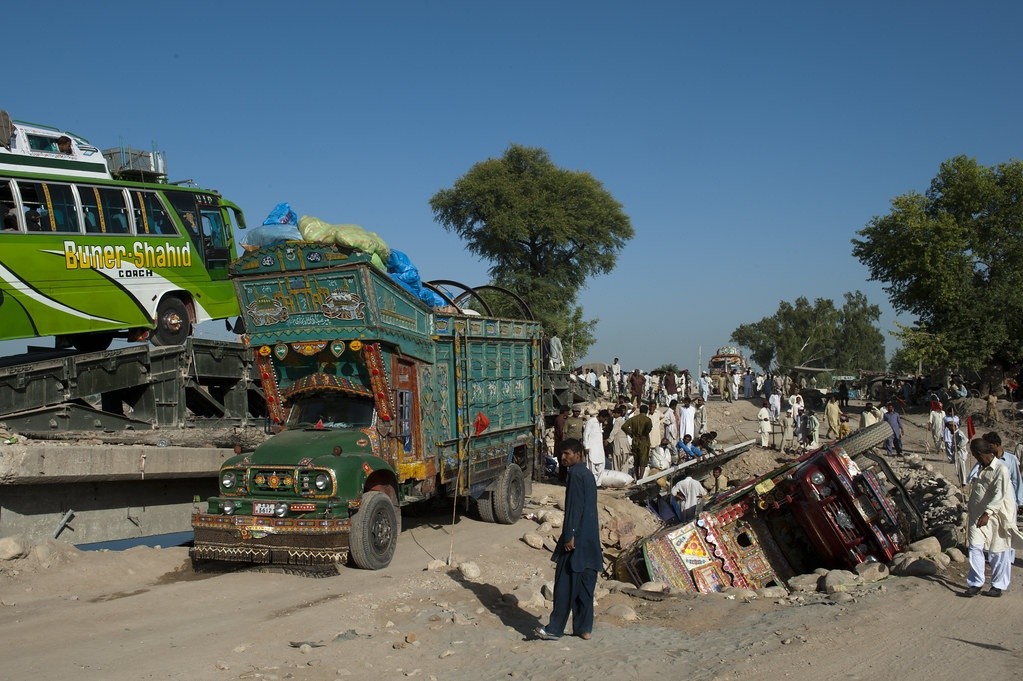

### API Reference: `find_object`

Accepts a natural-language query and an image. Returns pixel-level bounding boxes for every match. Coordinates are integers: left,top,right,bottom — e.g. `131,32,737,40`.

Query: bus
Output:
708,345,751,394
0,118,246,353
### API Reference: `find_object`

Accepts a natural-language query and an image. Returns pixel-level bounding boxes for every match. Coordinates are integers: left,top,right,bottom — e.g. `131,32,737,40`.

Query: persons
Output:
859,402,904,456
769,388,782,422
47,137,71,152
823,397,851,440
789,388,804,408
941,408,959,464
948,381,967,399
948,421,968,487
838,380,849,407
963,439,1023,598
1004,385,1014,402
928,402,946,453
554,357,734,513
985,390,999,422
717,367,816,403
26,206,41,231
544,331,565,370
533,438,603,640
968,432,1023,515
903,377,928,407
757,397,819,454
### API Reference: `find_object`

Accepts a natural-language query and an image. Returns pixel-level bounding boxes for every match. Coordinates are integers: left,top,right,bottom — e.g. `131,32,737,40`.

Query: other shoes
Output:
965,586,981,595
535,628,560,640
987,586,1002,596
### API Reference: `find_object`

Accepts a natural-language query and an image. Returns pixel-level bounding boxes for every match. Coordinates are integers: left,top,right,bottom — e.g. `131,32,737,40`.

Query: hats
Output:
573,405,582,412
559,405,570,412
787,409,792,413
798,407,804,410
763,400,769,404
702,371,707,373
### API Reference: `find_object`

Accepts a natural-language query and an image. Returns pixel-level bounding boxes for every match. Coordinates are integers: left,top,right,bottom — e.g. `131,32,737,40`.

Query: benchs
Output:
0,207,163,233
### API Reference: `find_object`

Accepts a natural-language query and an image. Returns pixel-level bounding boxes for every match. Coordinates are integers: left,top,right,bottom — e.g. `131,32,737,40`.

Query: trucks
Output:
186,236,542,578
611,421,970,598
